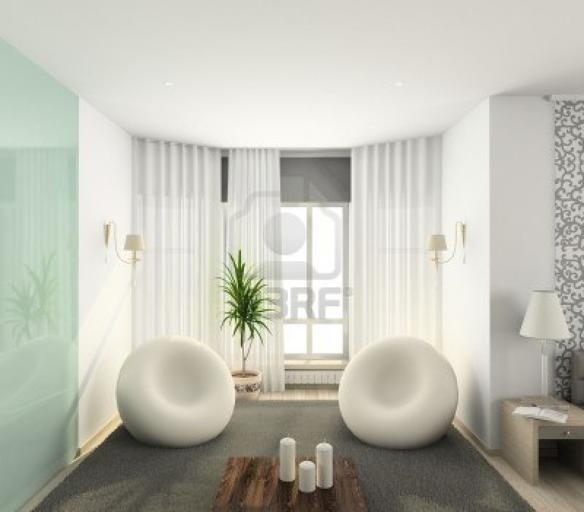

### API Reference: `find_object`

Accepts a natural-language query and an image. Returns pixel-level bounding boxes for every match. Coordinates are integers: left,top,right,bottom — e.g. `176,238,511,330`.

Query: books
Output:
510,405,569,426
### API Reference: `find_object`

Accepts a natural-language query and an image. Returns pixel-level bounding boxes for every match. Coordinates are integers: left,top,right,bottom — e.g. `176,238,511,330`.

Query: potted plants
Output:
217,249,281,403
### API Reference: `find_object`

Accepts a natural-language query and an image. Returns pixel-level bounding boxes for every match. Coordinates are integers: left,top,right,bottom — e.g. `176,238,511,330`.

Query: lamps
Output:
106,218,148,268
516,290,574,409
427,221,465,269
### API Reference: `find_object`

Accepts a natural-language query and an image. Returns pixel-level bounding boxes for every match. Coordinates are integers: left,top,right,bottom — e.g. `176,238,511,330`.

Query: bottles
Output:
299,460,319,494
279,433,298,484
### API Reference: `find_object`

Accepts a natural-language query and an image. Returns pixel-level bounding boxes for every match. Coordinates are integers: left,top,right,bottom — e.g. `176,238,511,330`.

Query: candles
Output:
298,460,316,493
277,437,296,482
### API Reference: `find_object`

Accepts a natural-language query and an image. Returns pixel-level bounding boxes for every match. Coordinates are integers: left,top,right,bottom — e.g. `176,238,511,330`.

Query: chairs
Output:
337,336,458,449
115,333,235,449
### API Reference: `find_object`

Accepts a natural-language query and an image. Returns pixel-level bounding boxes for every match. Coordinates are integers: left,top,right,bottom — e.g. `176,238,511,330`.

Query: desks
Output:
213,454,370,512
500,394,584,486
